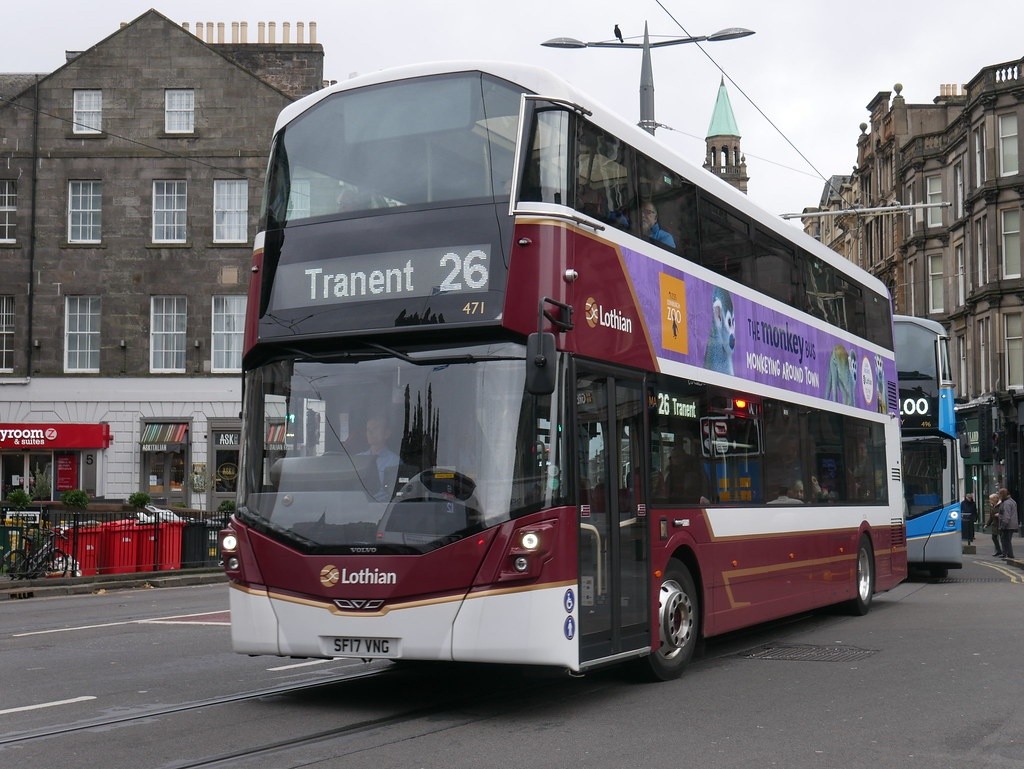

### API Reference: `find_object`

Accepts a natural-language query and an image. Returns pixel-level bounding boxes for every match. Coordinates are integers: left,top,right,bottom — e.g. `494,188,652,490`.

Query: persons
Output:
581,446,713,504
960,493,979,546
787,474,837,505
845,438,871,487
640,197,677,248
983,488,1019,561
349,410,406,490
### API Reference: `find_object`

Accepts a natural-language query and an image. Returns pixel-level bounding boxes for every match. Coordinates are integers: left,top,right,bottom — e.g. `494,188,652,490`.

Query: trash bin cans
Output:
99,519,141,576
201,518,224,568
46,528,70,579
66,526,103,576
156,520,187,571
134,519,166,573
182,517,207,568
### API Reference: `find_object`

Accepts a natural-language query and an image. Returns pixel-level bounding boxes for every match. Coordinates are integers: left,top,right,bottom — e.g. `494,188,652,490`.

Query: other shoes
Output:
999,554,1006,558
992,550,1002,556
1002,556,1014,561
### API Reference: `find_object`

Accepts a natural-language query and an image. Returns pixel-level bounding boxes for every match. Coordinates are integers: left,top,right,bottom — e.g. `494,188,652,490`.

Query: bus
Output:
891,313,972,571
217,57,909,681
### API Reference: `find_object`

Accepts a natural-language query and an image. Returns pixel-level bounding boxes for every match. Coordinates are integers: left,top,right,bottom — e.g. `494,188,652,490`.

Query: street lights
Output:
539,20,757,138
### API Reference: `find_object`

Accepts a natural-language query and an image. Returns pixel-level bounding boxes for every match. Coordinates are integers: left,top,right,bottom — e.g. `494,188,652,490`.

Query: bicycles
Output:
0,528,69,581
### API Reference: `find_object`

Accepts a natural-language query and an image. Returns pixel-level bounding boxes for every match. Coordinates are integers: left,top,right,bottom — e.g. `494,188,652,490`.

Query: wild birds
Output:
614,24,625,44
